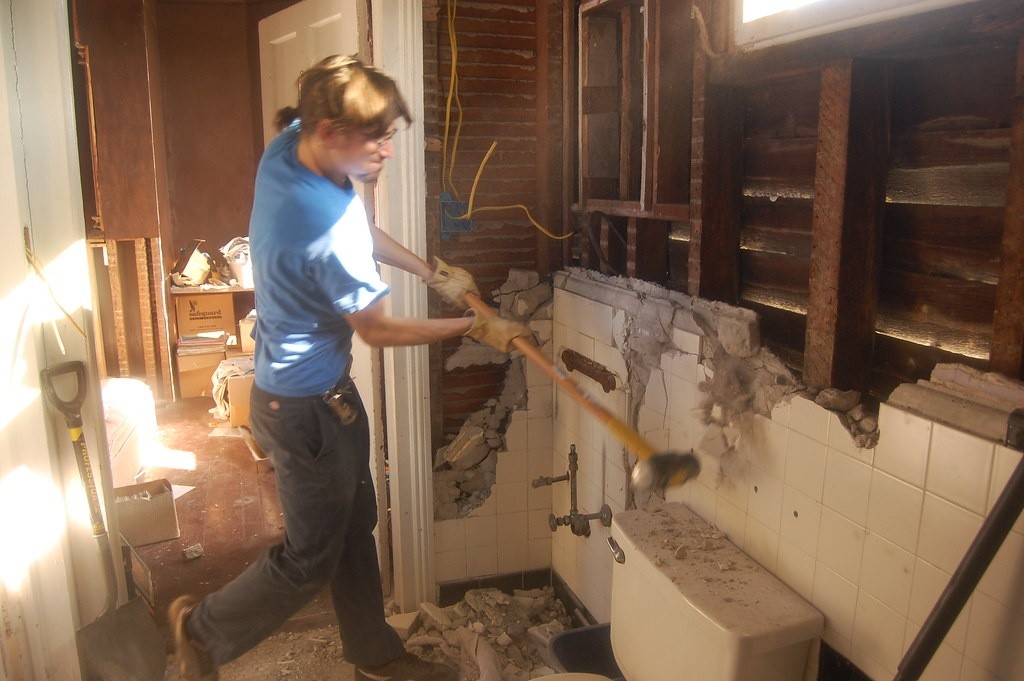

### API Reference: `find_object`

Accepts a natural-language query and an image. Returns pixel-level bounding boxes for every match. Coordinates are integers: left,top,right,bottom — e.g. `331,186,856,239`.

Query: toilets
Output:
528,500,827,680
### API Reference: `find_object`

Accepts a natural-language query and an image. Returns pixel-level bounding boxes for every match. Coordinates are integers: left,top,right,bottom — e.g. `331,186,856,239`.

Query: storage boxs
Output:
173,292,255,427
113,478,179,547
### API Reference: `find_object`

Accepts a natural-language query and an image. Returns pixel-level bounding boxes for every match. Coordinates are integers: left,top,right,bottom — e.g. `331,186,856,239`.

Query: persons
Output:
169,54,536,681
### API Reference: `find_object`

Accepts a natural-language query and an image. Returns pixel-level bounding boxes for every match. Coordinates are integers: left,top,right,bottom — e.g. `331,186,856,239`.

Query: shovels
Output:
40,360,169,681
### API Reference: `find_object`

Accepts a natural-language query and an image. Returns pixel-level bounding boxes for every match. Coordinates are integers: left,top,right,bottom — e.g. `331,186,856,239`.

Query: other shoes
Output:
354,649,457,681
168,595,219,681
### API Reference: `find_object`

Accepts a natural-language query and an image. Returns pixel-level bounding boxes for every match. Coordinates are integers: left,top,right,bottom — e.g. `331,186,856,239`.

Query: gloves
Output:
461,308,533,352
421,256,481,310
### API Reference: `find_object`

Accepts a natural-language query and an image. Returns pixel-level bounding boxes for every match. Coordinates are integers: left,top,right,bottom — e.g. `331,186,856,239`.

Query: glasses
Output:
337,121,399,147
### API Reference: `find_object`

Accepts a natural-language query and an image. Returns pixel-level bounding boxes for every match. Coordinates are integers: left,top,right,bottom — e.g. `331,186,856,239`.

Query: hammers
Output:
461,289,701,492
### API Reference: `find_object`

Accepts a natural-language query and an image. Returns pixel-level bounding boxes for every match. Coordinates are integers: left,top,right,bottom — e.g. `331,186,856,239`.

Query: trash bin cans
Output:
546,622,626,681
